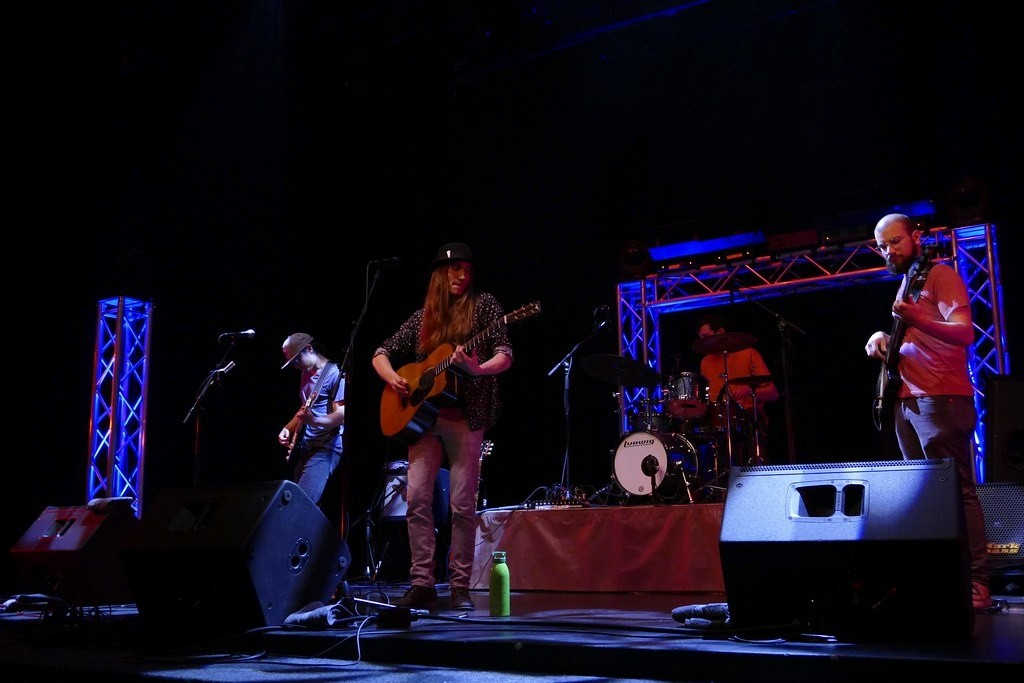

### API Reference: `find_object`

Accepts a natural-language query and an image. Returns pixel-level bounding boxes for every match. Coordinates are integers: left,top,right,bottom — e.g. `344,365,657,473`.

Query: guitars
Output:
284,391,317,470
379,299,543,446
873,244,939,433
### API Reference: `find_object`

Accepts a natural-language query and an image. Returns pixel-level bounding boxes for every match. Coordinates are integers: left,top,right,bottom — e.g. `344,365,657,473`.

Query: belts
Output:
429,400,468,409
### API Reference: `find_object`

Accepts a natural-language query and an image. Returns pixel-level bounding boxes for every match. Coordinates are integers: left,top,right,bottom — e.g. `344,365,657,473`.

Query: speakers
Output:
718,457,1024,643
9,479,351,629
377,461,453,579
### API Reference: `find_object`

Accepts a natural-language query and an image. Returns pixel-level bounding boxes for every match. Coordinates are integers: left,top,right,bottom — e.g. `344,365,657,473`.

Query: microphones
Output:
225,329,256,339
647,457,656,465
729,277,734,303
595,305,610,310
395,477,409,489
370,257,399,264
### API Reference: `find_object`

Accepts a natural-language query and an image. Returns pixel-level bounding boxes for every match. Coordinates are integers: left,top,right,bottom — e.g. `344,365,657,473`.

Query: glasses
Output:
288,349,304,368
874,232,909,250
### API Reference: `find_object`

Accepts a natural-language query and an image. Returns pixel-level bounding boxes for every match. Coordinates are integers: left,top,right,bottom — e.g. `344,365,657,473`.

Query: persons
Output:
864,214,993,608
279,333,345,504
697,317,779,429
372,243,514,610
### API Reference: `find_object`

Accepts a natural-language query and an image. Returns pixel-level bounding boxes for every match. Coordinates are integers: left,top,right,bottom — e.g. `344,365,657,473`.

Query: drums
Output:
668,371,710,418
700,399,739,434
633,412,673,433
609,429,701,498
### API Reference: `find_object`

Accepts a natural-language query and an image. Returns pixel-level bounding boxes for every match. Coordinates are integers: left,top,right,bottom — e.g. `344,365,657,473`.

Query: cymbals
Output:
579,354,662,387
691,332,758,354
729,375,779,385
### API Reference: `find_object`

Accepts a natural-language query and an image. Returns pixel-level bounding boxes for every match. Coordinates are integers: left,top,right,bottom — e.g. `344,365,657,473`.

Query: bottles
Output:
489,551,510,616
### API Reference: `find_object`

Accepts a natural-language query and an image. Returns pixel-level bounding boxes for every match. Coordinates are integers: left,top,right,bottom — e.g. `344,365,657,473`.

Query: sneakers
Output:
396,584,439,609
970,582,992,609
450,586,475,611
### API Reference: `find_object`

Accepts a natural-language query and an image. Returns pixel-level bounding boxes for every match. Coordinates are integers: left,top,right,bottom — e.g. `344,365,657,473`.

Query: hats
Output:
429,242,475,267
279,332,314,370
